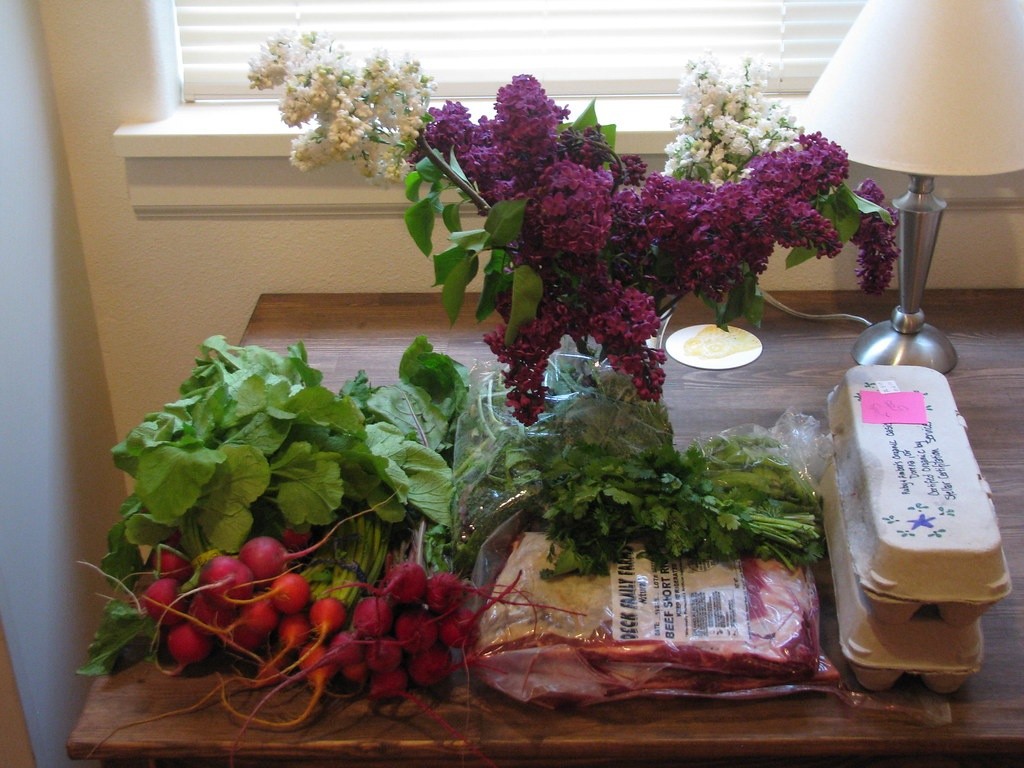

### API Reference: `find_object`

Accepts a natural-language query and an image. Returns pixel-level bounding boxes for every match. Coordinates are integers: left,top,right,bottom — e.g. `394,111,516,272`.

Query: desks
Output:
63,288,1024,766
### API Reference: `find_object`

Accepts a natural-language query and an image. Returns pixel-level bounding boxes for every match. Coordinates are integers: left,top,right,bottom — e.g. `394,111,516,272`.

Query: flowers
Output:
250,27,900,429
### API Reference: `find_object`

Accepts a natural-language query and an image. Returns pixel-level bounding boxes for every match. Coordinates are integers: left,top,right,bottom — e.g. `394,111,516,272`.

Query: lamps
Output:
795,0,1024,375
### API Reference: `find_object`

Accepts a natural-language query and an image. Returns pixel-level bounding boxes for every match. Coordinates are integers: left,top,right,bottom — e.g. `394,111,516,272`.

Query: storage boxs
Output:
826,365,1011,627
820,454,986,693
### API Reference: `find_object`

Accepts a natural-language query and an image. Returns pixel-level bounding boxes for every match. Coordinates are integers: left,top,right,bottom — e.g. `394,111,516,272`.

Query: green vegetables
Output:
73,337,825,676
